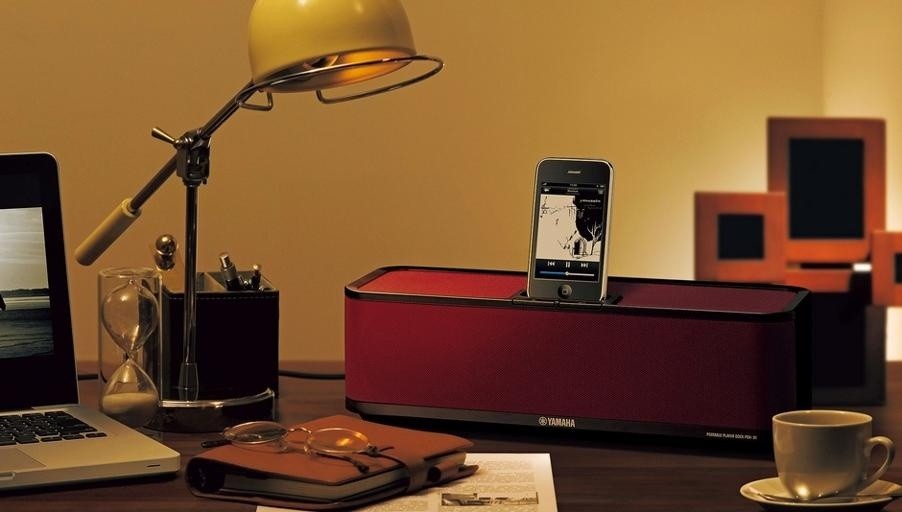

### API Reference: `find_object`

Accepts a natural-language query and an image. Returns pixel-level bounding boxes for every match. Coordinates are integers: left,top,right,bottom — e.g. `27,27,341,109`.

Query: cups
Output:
771,408,894,505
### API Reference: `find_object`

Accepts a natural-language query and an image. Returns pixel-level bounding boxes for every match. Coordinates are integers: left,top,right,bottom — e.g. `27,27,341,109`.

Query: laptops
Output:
0,150,181,491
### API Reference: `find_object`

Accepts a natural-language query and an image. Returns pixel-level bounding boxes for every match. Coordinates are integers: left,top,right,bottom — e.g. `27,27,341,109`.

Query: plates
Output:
739,475,902,512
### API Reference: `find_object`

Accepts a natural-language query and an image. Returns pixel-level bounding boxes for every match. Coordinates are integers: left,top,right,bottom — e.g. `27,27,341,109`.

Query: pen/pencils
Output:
201,429,283,448
219,252,262,291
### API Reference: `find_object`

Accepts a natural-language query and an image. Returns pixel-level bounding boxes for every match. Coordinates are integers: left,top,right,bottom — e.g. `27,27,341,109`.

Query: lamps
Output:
75,0,445,435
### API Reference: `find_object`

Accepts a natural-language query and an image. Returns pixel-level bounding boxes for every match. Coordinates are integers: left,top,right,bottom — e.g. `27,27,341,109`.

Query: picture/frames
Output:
693,116,901,306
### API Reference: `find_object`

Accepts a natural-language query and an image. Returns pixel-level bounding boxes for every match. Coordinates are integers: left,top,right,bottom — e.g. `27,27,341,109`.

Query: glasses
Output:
200,422,381,471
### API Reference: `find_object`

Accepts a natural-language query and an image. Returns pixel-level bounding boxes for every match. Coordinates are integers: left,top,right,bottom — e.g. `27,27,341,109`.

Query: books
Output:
182,412,479,510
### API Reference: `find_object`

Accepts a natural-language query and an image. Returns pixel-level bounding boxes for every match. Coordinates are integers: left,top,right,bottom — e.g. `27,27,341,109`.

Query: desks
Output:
2,357,900,510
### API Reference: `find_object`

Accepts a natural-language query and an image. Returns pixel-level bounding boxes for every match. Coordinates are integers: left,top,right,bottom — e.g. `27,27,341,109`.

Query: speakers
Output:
343,262,810,461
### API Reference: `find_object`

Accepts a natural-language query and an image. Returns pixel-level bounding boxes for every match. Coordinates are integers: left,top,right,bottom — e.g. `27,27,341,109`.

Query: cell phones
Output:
525,155,615,303
